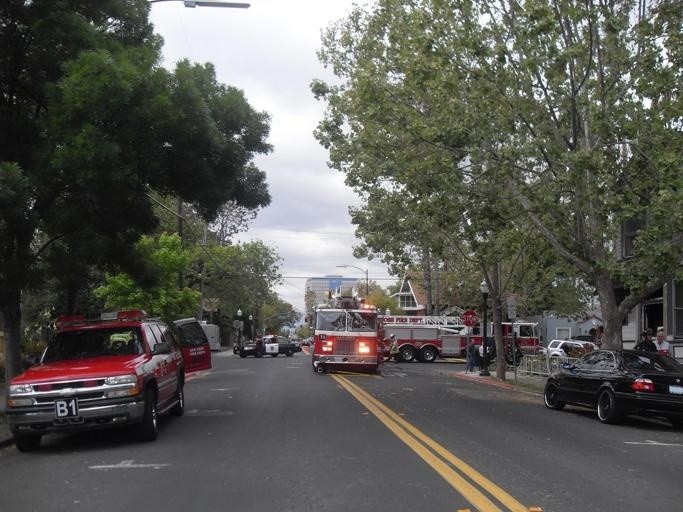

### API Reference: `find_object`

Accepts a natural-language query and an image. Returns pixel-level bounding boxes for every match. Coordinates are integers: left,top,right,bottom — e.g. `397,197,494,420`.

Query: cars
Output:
545,348,683,425
234,336,315,359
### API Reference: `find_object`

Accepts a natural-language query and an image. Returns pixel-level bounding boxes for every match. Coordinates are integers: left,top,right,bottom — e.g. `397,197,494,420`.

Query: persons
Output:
491,335,497,360
633,326,657,353
465,339,477,372
653,327,671,357
478,341,488,369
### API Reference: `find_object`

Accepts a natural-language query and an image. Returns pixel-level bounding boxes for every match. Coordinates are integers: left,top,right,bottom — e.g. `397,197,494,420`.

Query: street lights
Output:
336,265,369,298
236,307,243,346
147,1,250,12
478,276,491,377
249,315,255,340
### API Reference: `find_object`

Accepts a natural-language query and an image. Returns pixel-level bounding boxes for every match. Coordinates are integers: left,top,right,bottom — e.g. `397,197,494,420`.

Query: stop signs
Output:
462,309,478,327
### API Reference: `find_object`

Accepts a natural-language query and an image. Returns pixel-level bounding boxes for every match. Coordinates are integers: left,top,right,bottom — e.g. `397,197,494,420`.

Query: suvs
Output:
8,306,215,452
537,338,600,362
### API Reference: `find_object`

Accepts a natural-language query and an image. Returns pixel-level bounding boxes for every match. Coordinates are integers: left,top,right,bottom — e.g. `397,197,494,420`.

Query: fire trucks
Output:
377,312,542,367
312,285,389,374
